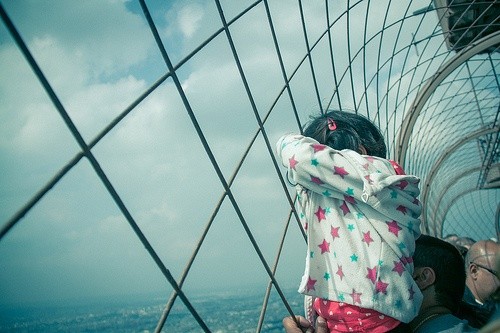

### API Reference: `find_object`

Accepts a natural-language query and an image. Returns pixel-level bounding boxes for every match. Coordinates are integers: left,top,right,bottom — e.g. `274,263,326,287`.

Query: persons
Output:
275,111,422,333
442,233,499,333
280,233,485,333
